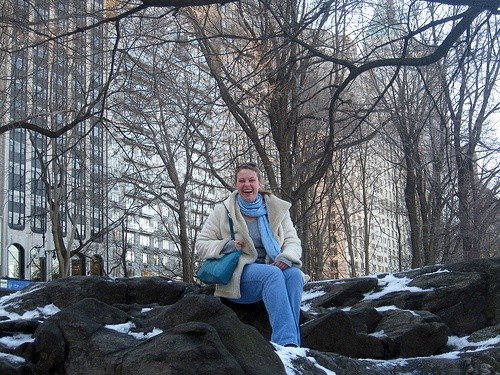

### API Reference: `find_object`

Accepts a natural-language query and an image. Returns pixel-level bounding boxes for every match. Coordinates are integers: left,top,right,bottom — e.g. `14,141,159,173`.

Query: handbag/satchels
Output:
198,200,240,286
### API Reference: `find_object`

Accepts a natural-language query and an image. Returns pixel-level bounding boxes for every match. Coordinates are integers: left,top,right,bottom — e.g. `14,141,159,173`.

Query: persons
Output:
196,161,310,348
